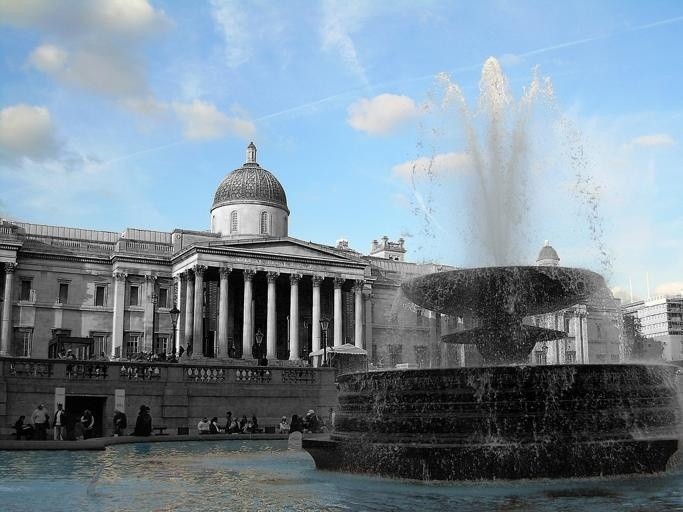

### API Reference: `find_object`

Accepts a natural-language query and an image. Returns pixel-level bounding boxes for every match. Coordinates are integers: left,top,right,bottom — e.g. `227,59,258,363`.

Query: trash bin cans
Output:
178,427,189,435
265,427,275,433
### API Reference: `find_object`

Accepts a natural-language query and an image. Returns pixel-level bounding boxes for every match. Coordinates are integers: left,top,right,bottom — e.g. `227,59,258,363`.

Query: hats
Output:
307,410,316,416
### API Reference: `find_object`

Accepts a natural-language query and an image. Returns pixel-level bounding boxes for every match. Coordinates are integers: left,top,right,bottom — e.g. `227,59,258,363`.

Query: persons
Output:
90,352,110,361
197,411,258,434
15,400,95,440
112,409,127,436
125,350,163,361
66,349,76,359
279,408,335,434
179,343,192,357
130,404,152,436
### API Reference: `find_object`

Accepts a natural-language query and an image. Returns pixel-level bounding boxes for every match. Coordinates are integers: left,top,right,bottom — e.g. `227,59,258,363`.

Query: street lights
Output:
319,314,331,366
169,304,180,357
254,328,263,363
150,293,157,355
574,300,589,365
542,342,548,365
